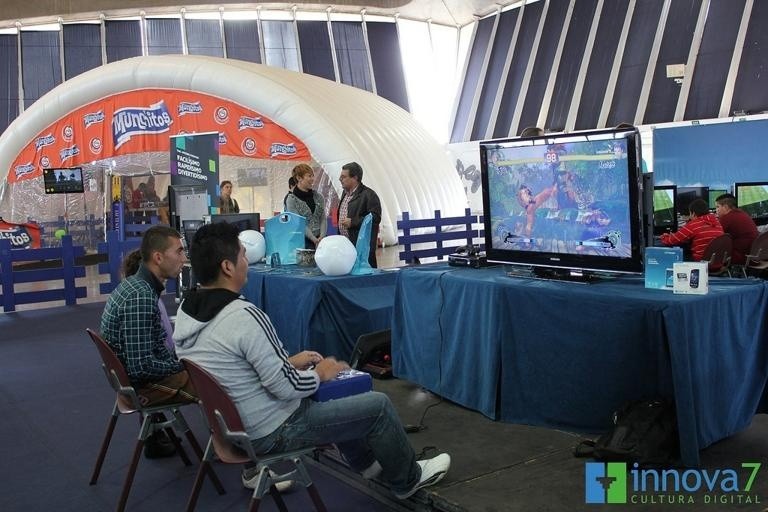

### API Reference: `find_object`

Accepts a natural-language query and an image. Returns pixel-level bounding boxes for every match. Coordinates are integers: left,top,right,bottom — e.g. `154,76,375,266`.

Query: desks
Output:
388,259,766,473
237,266,397,367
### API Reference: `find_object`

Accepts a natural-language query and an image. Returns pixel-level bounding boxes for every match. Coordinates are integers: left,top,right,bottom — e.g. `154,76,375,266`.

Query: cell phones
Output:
690,269,699,288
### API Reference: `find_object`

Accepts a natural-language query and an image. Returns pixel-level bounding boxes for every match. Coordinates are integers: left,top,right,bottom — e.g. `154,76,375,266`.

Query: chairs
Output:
735,229,768,279
84,326,227,511
181,357,330,512
698,232,736,279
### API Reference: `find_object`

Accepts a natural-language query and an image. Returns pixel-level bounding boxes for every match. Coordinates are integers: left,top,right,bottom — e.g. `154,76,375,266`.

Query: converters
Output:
404,423,419,433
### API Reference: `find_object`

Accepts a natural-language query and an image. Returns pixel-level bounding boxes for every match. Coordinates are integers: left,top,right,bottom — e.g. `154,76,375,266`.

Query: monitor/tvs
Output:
43,167,85,194
709,190,727,210
211,214,260,238
734,182,768,226
677,186,709,220
478,127,644,285
653,185,678,238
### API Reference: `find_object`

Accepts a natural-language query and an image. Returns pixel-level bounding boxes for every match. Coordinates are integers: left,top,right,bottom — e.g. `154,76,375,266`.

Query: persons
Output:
69,173,75,190
120,248,178,457
132,183,145,236
287,164,326,249
173,223,452,499
218,180,238,214
284,176,296,211
57,172,67,190
660,201,724,272
335,162,381,268
98,225,199,408
144,176,161,224
715,194,758,266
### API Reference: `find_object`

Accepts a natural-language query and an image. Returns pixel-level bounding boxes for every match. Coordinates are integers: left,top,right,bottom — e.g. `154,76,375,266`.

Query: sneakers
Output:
361,456,383,480
241,467,296,492
394,453,450,499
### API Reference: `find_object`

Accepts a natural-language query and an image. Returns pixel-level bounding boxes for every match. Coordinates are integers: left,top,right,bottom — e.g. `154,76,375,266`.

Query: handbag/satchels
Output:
350,212,375,275
261,213,308,266
